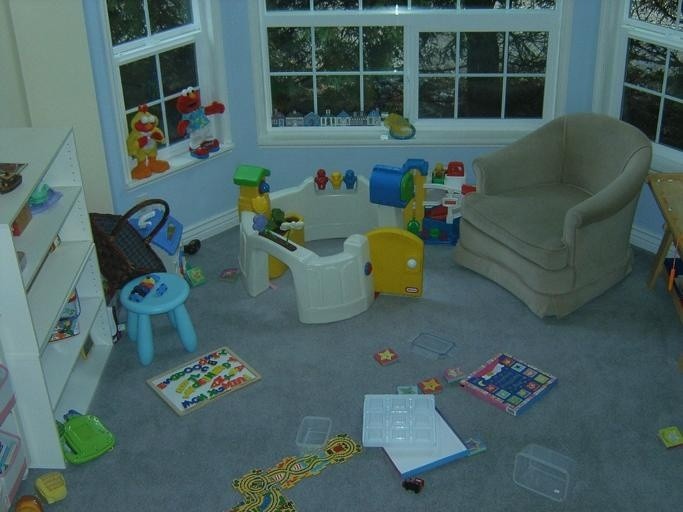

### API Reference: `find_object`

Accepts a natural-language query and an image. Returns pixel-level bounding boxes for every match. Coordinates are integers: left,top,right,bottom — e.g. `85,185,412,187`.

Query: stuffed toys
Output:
174,85,224,158
126,105,168,181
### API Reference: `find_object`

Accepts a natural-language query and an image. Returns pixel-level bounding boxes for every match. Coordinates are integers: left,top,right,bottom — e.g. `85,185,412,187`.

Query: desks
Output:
646,172,683,322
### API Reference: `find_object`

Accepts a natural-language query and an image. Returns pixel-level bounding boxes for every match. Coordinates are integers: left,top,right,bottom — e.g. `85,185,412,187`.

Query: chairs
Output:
453,112,653,321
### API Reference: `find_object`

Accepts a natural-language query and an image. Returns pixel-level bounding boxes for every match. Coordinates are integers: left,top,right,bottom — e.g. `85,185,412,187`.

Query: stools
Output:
120,272,199,366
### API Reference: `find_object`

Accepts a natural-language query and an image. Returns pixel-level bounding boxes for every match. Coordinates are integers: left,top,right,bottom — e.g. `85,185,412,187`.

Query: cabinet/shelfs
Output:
1,127,115,470
